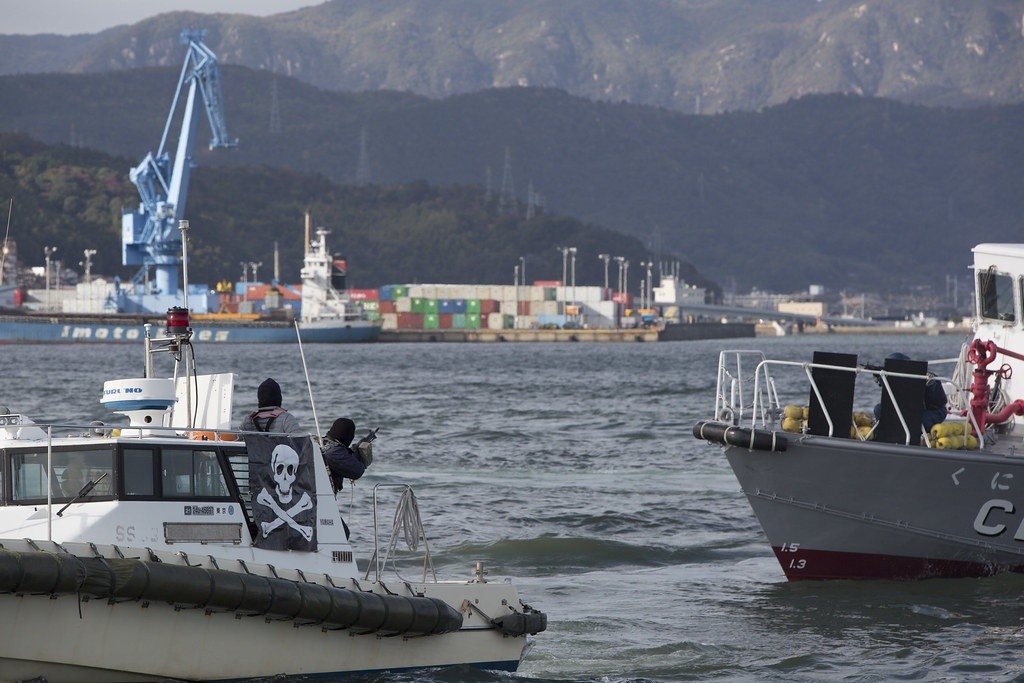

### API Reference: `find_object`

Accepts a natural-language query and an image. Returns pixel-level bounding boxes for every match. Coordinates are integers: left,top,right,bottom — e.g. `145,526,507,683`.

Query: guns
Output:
352,427,380,451
859,361,884,386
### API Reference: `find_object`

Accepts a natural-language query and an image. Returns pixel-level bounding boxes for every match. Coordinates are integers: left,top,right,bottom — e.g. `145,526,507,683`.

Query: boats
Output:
1,307,546,682
1,229,384,342
691,242,1024,580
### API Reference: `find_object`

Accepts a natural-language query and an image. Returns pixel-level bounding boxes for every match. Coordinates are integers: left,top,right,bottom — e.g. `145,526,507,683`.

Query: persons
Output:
318,418,364,541
237,377,302,433
874,353,948,432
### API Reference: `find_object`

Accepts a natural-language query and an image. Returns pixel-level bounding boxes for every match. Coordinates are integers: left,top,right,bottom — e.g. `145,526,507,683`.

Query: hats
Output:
258,378,282,407
326,417,355,448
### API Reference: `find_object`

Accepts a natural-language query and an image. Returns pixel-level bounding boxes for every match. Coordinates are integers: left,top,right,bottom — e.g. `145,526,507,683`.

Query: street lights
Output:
514,247,680,327
